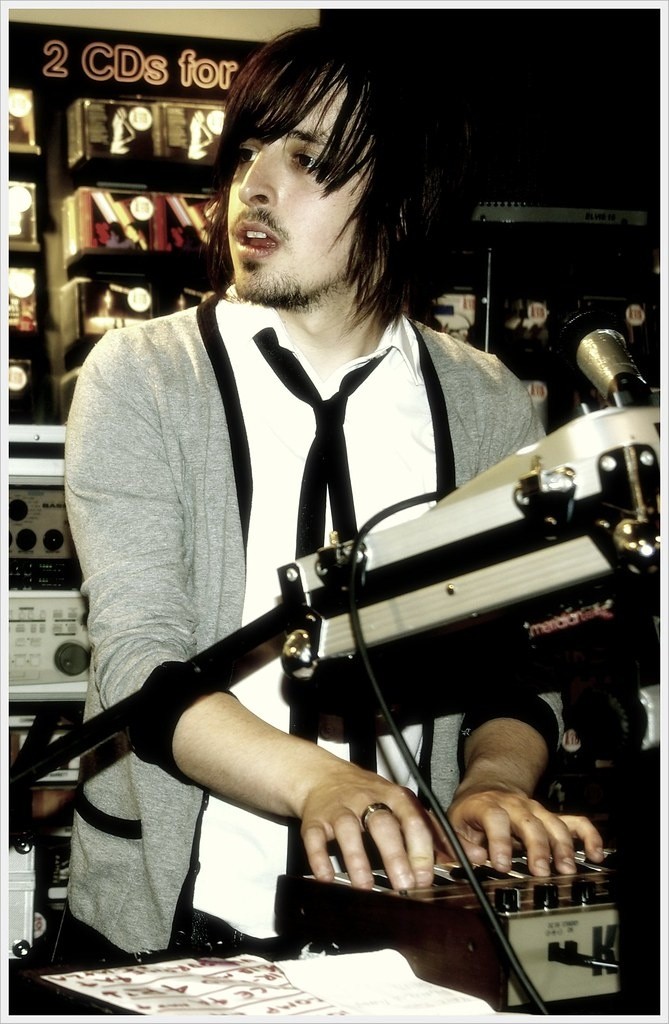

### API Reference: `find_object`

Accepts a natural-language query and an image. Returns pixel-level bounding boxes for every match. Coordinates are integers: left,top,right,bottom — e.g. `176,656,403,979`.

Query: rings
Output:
361,803,392,833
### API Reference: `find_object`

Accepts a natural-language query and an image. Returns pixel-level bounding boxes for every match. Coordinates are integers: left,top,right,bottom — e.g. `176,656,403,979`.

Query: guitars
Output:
0,442,661,801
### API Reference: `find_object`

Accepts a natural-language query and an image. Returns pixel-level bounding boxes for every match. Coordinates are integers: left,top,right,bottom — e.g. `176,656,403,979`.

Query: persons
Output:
51,26,605,957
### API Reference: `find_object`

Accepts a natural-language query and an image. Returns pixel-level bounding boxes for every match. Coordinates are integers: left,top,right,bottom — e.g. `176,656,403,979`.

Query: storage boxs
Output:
8,86,245,419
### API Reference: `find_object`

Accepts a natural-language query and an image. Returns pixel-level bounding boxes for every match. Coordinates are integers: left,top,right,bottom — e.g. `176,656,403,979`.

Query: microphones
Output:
558,310,652,408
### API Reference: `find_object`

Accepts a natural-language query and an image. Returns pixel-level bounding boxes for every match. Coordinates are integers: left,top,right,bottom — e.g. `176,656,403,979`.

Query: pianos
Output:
268,834,659,1016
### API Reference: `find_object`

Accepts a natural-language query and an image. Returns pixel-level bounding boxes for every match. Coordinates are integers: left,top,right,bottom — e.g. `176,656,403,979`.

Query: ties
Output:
251,328,389,877
